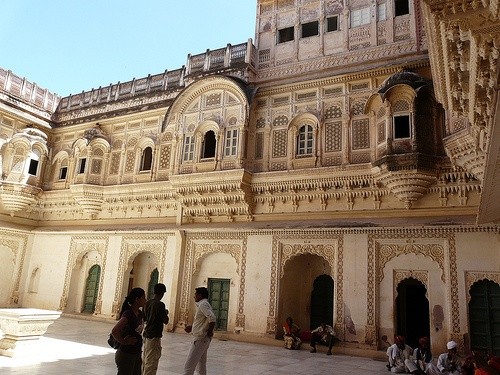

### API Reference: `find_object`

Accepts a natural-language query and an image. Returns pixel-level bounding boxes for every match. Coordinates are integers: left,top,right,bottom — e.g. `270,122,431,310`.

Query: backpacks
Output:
107,310,131,349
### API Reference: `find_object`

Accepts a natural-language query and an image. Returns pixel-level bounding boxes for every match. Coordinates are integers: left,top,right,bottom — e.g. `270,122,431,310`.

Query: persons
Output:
184,287,216,375
310,322,336,355
111,287,147,375
386,335,500,375
142,283,169,375
283,317,301,350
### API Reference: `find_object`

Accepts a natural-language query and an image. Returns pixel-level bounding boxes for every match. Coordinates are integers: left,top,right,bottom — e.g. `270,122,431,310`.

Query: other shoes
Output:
327,351,332,355
310,350,316,353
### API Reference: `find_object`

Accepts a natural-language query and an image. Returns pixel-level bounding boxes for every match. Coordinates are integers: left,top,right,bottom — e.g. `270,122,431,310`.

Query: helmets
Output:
395,335,405,342
419,337,429,346
490,356,500,371
447,341,457,349
